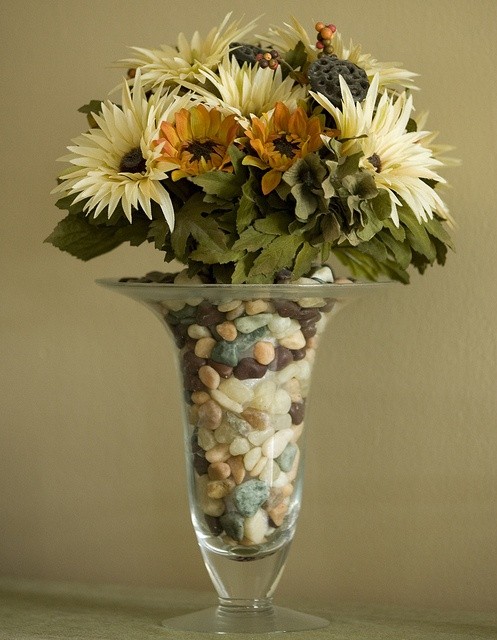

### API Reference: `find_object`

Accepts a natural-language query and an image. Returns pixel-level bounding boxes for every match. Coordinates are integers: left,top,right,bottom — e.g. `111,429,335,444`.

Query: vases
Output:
90,277,388,635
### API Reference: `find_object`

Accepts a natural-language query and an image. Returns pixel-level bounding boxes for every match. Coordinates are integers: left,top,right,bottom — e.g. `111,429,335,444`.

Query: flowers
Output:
42,7,456,285
